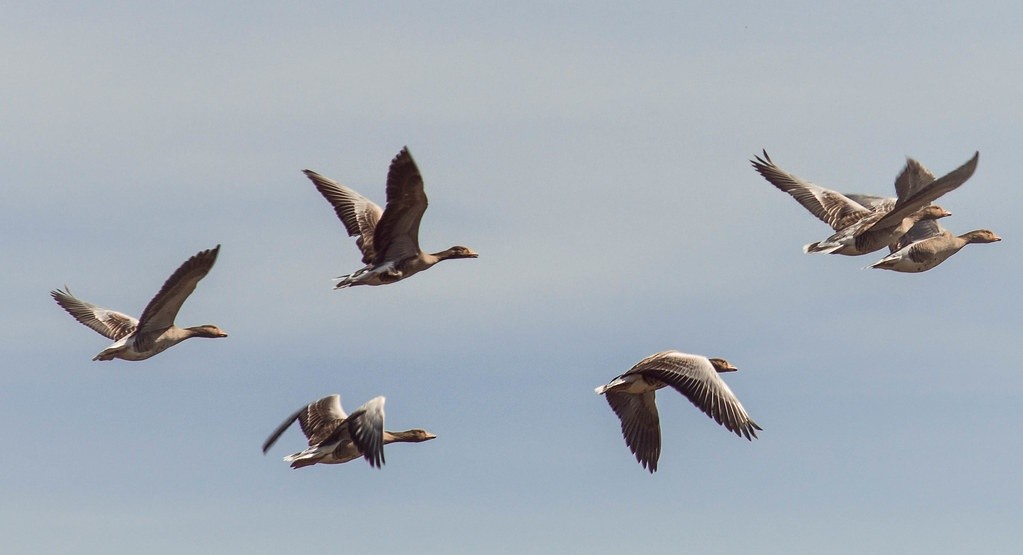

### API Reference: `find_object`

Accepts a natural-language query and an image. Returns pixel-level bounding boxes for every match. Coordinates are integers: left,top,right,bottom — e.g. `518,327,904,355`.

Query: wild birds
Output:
843,194,1002,273
51,244,228,361
301,145,480,291
749,148,979,257
261,394,436,469
594,350,764,475
